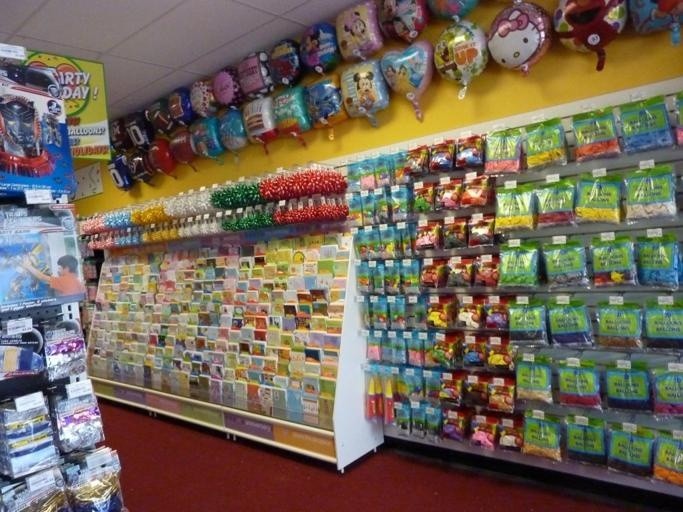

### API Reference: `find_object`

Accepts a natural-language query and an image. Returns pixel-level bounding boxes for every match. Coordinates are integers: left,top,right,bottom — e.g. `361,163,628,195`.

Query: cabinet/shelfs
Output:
84,233,386,478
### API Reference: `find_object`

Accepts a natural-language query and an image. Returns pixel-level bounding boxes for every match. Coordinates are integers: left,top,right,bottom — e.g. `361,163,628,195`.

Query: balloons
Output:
486,2,554,77
237,50,278,155
552,1,628,72
630,1,682,44
190,78,225,166
335,4,389,128
269,38,312,148
378,1,433,123
213,66,250,163
106,119,134,190
127,110,157,188
168,85,199,174
145,98,178,180
300,22,349,141
428,2,489,101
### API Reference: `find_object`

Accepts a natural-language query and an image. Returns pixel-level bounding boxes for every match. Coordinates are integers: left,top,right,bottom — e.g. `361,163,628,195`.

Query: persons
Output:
22,255,81,296
